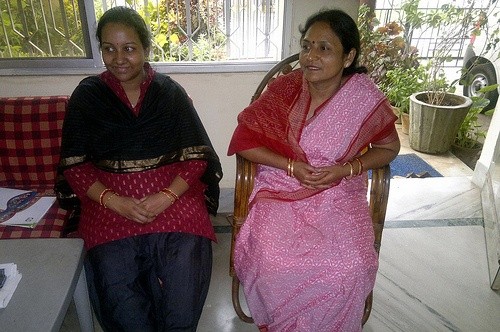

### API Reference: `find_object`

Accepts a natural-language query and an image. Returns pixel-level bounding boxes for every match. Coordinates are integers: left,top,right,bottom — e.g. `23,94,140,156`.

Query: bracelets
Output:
99,189,119,208
353,157,364,177
287,157,294,178
159,188,180,205
343,161,353,181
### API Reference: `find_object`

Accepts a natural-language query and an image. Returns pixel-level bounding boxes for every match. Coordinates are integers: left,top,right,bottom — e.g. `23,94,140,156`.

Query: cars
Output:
459,0,500,114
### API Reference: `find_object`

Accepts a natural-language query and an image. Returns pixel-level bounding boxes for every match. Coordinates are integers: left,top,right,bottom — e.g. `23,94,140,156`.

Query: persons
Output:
58,6,223,332
227,11,400,332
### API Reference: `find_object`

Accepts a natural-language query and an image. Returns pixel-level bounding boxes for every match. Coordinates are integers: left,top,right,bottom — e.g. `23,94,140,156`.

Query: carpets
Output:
367,153,444,180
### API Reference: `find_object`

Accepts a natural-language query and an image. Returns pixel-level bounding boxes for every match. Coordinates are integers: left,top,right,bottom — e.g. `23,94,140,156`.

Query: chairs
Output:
227,51,392,328
53,133,223,332
0,95,80,240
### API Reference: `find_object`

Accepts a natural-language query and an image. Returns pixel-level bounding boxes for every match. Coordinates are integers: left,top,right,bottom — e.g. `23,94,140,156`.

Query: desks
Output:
0,238,96,332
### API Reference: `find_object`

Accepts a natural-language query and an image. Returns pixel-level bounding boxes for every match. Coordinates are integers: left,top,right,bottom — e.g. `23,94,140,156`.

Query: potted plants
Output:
357,0,500,155
